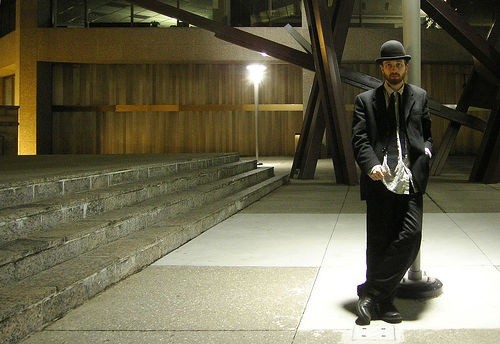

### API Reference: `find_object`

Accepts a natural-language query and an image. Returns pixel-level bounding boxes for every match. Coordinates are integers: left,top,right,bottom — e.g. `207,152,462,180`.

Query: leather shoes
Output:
380,303,403,323
355,285,377,325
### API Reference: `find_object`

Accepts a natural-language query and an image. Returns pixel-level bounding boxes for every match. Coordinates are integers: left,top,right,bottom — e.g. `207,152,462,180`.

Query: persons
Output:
351,40,432,325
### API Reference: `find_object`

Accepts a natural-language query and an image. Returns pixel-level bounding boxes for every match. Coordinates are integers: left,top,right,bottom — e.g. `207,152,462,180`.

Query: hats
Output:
375,40,412,63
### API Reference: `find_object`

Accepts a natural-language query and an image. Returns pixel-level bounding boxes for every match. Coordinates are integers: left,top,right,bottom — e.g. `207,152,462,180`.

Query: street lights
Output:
246,64,268,165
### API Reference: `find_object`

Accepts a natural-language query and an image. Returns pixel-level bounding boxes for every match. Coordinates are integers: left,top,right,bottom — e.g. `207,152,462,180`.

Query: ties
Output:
386,92,398,174
